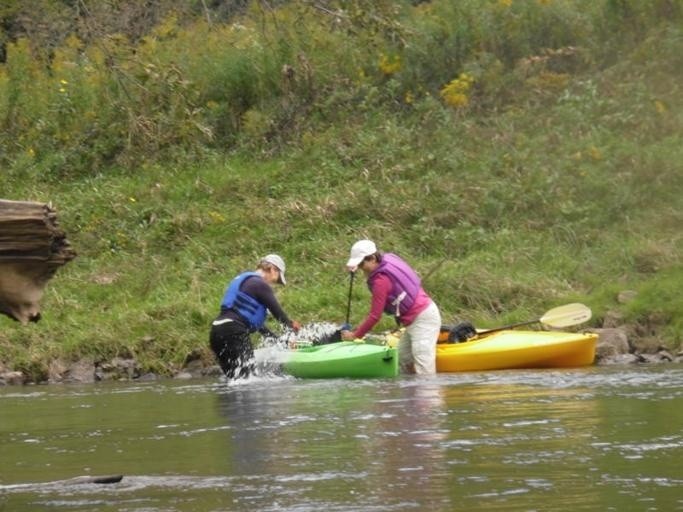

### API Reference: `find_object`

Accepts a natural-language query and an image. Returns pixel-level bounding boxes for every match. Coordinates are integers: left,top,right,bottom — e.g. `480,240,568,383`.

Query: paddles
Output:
476,303,592,335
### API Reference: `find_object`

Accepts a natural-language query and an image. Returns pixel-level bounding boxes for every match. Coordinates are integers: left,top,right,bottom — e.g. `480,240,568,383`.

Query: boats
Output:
378,320,599,375
260,327,399,384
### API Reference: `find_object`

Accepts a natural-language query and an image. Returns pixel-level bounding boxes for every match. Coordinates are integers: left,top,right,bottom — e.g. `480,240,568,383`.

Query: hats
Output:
262,252,289,287
345,239,377,268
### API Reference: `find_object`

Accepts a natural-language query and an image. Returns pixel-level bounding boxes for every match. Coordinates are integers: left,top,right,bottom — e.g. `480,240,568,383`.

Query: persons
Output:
339,239,441,377
209,253,302,381
